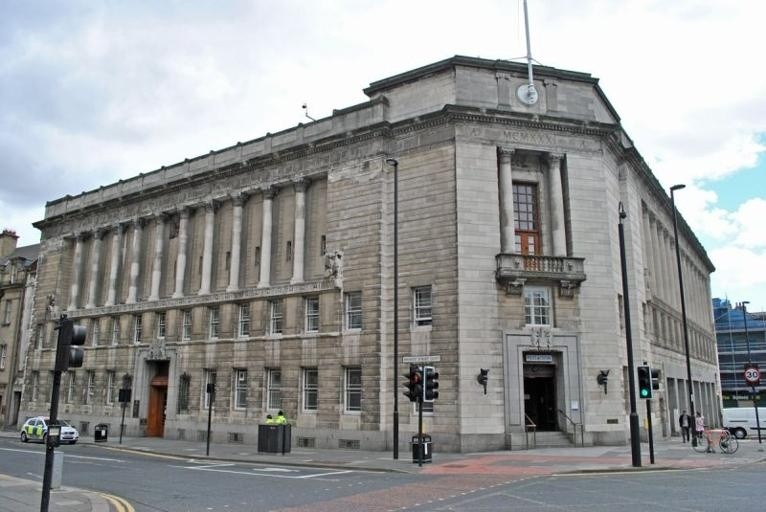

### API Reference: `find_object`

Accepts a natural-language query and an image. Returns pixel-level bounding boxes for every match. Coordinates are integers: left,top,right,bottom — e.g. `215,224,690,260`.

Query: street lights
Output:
386,158,398,460
670,185,697,447
742,302,761,443
618,202,642,466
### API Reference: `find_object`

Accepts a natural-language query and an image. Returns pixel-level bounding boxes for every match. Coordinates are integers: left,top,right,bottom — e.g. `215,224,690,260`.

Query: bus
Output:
722,386,766,439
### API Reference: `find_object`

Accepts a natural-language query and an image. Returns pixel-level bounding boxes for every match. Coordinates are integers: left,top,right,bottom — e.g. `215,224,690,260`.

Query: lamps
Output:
597,368,609,395
478,368,489,395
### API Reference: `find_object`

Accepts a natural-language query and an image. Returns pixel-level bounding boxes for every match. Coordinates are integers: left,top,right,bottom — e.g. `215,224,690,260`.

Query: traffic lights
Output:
403,364,439,403
55,319,86,371
637,366,661,398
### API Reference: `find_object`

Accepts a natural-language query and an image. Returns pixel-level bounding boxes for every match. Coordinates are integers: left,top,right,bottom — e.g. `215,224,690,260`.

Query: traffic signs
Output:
744,363,760,387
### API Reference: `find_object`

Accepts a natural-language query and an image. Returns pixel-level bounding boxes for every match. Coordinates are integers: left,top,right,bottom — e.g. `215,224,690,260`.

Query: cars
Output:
20,416,80,444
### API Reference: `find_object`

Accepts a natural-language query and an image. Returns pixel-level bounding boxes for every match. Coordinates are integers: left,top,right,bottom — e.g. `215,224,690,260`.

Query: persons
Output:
679,410,691,443
266,415,275,425
276,411,286,424
696,412,704,444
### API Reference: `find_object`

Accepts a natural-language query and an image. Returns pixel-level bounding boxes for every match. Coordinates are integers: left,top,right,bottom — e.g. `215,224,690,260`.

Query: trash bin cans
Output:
95,424,108,442
41,451,64,489
412,435,433,464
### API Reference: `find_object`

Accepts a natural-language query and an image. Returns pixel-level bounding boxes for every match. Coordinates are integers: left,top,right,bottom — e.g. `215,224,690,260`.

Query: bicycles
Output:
692,424,739,454
